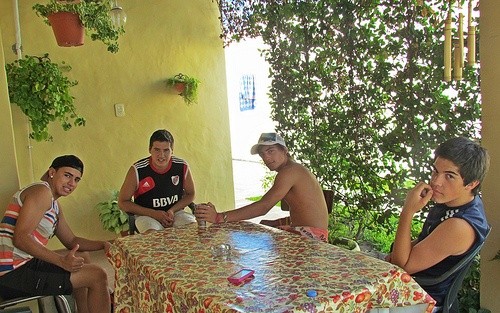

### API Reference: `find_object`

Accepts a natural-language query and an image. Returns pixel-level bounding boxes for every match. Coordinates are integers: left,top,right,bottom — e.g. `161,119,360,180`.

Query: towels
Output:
240,75,256,111
229,68,242,92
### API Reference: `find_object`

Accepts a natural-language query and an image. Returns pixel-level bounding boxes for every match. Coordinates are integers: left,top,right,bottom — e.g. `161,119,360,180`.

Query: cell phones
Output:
227,268,255,283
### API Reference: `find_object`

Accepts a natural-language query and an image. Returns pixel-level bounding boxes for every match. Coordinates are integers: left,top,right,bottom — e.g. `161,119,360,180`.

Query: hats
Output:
40,155,84,181
250,132,288,155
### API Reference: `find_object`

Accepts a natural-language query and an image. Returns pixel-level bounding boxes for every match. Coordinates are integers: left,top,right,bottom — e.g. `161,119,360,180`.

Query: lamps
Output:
110,0,126,25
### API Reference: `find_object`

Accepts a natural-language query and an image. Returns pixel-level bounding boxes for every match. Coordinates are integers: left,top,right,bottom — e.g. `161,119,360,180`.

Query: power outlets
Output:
115,104,125,119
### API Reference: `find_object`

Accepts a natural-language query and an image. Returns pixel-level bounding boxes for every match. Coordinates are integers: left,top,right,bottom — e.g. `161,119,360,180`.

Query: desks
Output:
104,221,436,313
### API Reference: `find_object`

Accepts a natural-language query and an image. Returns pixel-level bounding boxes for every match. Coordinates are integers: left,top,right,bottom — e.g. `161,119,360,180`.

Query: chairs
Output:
409,223,493,313
0,285,72,313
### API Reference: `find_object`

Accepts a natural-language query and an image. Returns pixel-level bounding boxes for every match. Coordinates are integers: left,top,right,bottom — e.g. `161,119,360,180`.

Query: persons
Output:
385,137,490,313
0,155,111,313
118,129,197,235
194,133,328,243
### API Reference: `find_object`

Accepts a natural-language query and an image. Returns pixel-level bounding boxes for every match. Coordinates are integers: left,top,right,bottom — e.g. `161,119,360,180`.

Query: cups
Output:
211,243,230,261
194,203,210,227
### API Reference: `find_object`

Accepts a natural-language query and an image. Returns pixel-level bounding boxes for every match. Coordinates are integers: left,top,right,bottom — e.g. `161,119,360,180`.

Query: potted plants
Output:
33,0,125,53
97,190,134,238
167,73,200,105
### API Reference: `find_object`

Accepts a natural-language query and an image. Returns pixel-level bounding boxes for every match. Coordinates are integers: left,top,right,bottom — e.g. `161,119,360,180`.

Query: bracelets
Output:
216,213,220,224
222,212,228,223
